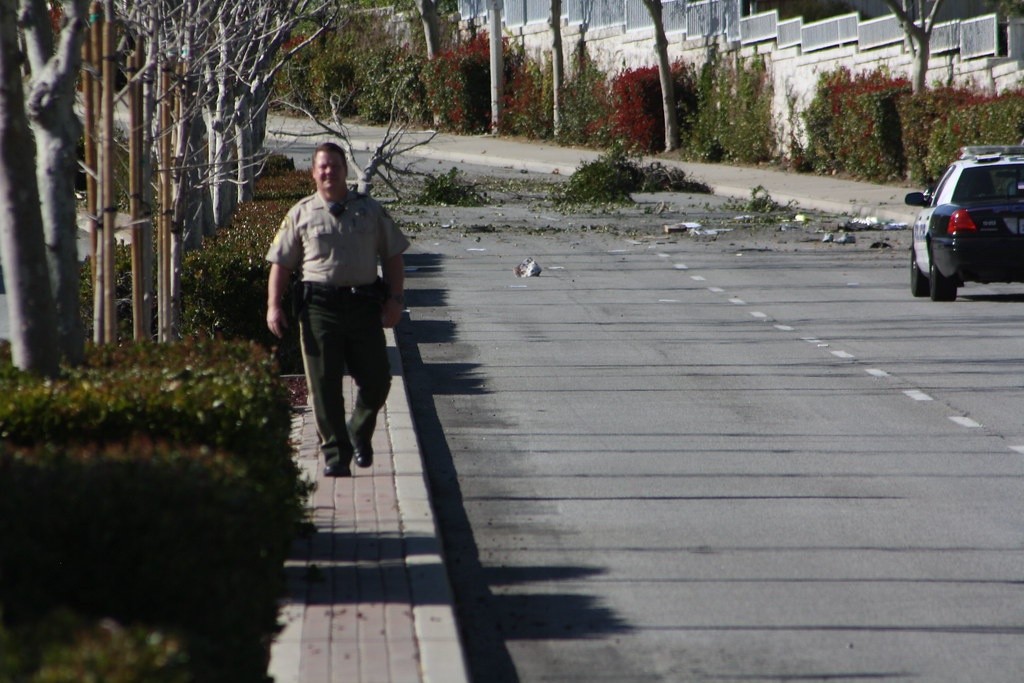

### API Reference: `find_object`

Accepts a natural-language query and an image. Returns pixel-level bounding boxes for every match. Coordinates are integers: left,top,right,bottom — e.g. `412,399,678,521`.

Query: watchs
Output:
393,293,406,304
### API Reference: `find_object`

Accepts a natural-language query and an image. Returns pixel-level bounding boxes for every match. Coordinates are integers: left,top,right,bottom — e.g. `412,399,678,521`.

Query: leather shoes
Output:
324,462,351,476
346,420,373,467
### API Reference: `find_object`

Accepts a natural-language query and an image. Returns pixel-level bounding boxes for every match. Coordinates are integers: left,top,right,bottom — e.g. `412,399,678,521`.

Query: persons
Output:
264,144,410,476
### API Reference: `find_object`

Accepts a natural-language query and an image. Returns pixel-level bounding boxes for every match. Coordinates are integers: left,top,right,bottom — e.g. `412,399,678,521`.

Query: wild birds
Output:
821,233,834,247
834,232,849,246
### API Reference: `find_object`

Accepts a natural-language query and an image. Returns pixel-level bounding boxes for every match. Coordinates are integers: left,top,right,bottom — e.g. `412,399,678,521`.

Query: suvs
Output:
904,152,1024,303
